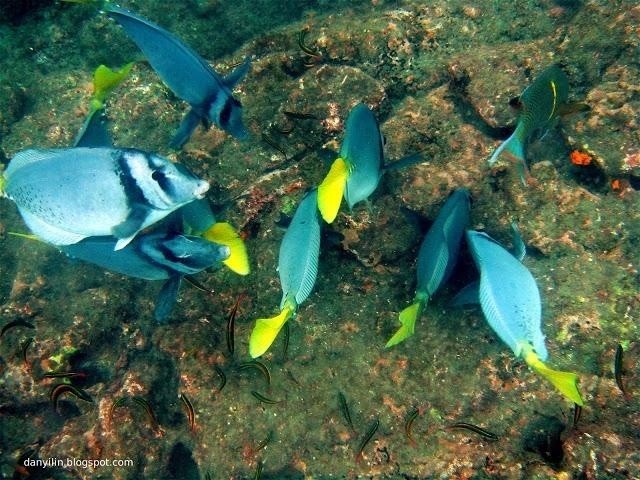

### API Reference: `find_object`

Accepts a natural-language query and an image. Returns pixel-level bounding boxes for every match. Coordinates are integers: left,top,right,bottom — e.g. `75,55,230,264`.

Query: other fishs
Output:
0,62,325,437
